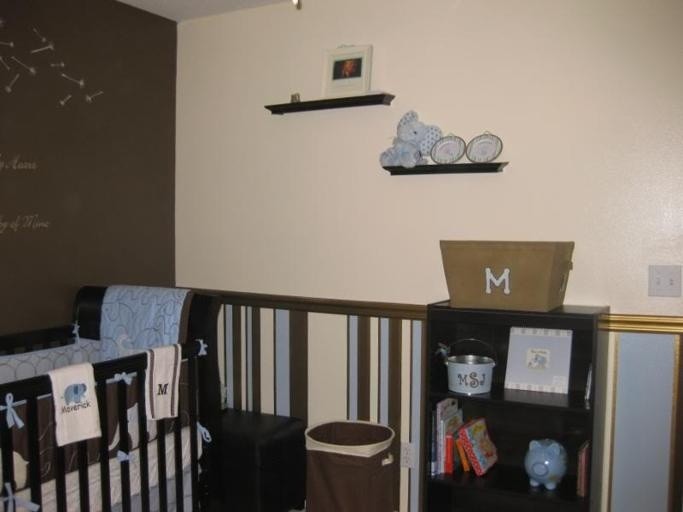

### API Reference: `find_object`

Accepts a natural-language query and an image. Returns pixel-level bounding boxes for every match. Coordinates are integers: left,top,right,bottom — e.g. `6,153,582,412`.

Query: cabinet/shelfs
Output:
417,296,610,512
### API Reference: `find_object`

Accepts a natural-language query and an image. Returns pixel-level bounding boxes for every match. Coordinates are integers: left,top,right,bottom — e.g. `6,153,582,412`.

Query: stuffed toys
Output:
379,109,441,168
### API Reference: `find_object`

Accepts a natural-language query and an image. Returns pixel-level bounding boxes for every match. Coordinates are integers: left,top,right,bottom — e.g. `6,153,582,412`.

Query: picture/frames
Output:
324,46,371,95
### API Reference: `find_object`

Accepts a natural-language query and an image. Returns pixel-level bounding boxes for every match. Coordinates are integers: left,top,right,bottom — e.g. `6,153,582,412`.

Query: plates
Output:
430,136,466,165
465,134,503,163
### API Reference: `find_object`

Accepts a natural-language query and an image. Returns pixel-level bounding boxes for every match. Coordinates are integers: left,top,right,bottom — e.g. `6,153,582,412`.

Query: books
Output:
576,437,589,497
430,397,497,477
506,327,572,393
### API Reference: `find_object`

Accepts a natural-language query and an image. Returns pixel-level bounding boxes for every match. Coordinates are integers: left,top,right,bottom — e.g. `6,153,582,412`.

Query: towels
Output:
43,361,103,446
138,342,183,423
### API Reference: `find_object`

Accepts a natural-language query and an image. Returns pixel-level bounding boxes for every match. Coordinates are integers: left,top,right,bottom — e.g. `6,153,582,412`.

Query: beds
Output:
0,282,227,512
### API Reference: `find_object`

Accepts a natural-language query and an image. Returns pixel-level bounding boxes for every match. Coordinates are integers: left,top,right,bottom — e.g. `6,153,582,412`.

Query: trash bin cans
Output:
304,420,398,512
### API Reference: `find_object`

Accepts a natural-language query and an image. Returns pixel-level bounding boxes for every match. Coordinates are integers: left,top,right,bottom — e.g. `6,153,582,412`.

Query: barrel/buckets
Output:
440,339,498,394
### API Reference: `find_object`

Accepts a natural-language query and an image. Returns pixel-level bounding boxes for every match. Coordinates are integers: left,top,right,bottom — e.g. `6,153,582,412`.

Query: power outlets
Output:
647,263,683,298
